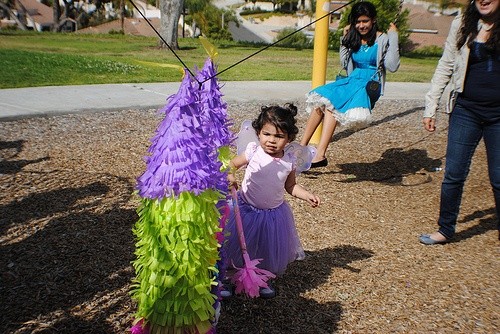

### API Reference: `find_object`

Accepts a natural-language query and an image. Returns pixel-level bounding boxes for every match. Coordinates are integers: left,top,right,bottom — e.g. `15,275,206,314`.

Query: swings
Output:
336,1,404,96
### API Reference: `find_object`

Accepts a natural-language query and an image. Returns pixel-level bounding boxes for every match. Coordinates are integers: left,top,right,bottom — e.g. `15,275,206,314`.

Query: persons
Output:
220,102,321,298
420,0,500,245
295,1,401,168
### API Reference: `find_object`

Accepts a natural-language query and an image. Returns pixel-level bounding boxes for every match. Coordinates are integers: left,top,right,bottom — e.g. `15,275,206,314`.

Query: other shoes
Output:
419,231,450,244
261,281,275,298
219,290,231,297
311,158,328,168
